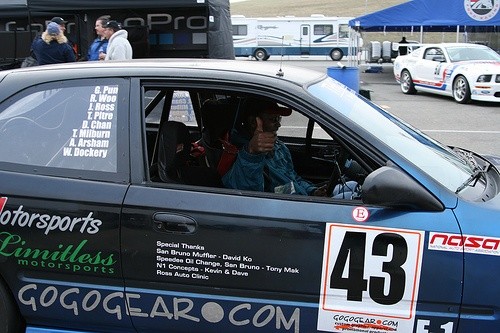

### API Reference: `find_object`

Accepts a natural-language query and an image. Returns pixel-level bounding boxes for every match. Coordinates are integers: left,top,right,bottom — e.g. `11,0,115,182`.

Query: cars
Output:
394,43,500,103
0,58,500,333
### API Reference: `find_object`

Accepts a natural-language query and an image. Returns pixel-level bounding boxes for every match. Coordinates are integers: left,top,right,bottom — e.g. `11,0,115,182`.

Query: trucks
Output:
0,0,236,70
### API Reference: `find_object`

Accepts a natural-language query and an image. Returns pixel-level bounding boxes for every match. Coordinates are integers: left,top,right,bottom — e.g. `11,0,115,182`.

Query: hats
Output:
47,22,60,36
247,98,293,118
51,17,68,24
102,21,122,30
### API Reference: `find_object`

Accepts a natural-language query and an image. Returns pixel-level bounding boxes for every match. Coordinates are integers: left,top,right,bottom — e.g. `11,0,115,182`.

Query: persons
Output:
216,94,360,201
30,22,76,65
87,15,112,61
32,17,78,65
99,20,133,61
399,37,407,55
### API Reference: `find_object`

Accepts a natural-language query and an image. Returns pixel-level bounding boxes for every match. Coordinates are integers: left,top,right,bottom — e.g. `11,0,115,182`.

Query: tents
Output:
347,0,499,68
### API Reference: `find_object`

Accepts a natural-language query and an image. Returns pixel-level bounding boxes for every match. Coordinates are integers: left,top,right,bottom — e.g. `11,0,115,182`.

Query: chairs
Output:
159,120,200,186
201,96,249,185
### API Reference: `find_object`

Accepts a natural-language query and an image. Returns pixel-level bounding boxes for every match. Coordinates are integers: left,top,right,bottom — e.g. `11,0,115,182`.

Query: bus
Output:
231,14,364,61
